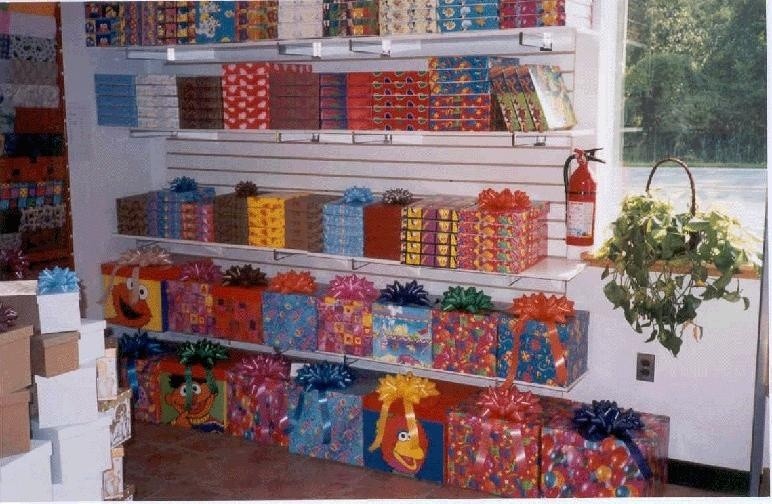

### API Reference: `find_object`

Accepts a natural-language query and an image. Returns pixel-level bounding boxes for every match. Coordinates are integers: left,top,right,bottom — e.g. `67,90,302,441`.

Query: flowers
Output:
593,186,764,358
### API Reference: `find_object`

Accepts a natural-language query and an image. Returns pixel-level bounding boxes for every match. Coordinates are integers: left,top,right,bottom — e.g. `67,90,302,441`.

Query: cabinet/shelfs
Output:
100,22,593,399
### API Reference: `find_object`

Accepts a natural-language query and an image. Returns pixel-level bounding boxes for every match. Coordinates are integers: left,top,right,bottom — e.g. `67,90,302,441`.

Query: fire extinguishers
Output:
564,148,606,247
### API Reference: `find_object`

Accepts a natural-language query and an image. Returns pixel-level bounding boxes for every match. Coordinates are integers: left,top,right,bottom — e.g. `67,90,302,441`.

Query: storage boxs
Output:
162,270,227,337
158,347,262,436
539,405,669,498
227,355,324,451
317,288,383,358
370,291,445,368
452,389,574,499
432,301,516,376
216,280,266,347
288,367,394,468
266,283,328,355
365,382,477,489
101,251,213,333
496,307,591,389
116,339,192,426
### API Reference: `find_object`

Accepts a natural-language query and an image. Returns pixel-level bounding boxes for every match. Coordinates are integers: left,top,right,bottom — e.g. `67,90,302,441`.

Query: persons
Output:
164,374,224,435
